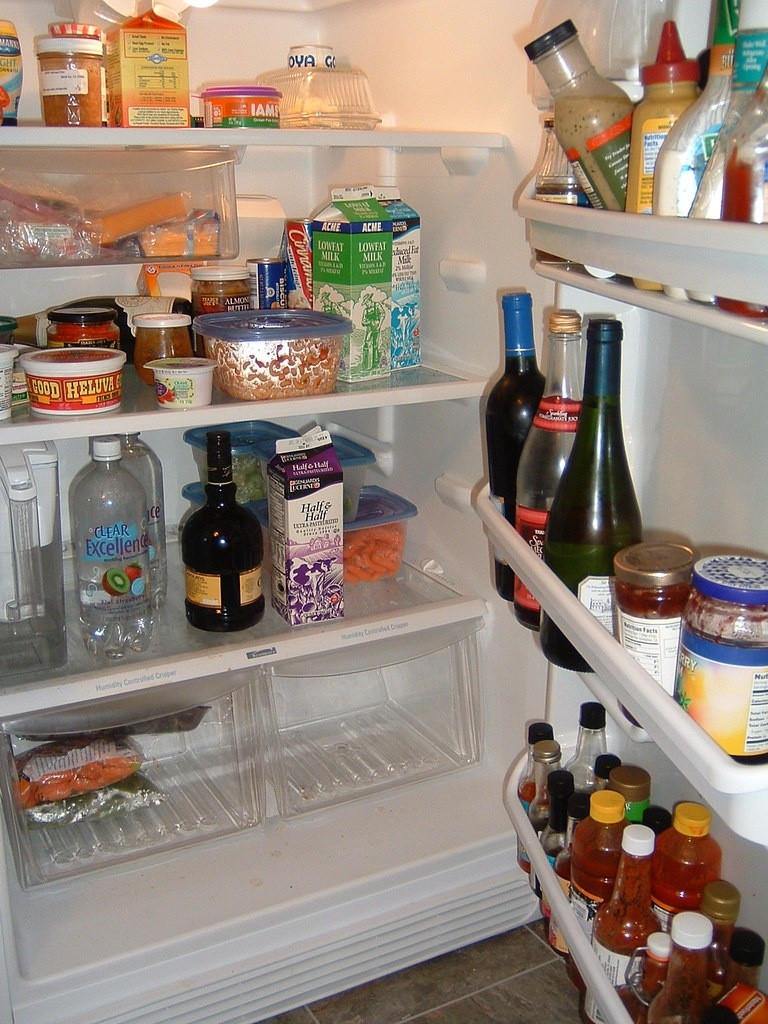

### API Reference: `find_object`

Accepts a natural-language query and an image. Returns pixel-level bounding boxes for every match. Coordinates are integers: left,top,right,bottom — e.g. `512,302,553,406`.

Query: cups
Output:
132,313,192,386
0,343,20,421
0,19,24,127
0,315,18,345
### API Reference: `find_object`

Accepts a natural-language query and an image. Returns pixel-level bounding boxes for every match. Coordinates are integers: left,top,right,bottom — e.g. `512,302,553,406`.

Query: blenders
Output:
0,440,68,679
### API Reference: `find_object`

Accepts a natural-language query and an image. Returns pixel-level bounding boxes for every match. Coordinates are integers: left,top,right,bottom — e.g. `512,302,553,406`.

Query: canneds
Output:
675,556,768,765
37,37,103,127
190,266,249,318
283,217,314,309
612,540,694,695
204,87,282,127
246,258,290,309
534,118,593,265
46,307,120,350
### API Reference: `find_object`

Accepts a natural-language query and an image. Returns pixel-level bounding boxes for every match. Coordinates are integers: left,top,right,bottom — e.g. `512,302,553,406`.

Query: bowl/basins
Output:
191,306,355,401
142,356,218,410
18,346,127,416
181,420,420,584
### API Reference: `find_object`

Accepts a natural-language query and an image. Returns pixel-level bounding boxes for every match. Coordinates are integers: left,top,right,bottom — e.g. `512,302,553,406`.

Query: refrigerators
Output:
1,0,767,1022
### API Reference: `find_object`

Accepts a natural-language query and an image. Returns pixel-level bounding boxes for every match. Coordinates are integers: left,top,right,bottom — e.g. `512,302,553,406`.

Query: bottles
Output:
540,319,645,675
624,19,703,292
516,701,768,1024
652,0,768,318
111,433,168,609
514,308,588,631
484,291,564,603
180,431,266,633
71,435,154,662
523,19,635,212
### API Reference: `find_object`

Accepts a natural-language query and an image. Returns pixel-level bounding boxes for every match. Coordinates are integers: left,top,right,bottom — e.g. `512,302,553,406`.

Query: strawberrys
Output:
125,562,142,583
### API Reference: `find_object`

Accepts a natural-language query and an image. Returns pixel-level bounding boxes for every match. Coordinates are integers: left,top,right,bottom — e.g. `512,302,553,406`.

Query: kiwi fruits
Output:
102,567,130,596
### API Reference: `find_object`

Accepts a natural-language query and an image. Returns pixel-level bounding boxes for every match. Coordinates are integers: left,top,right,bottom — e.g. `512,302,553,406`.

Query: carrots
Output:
342,521,403,581
